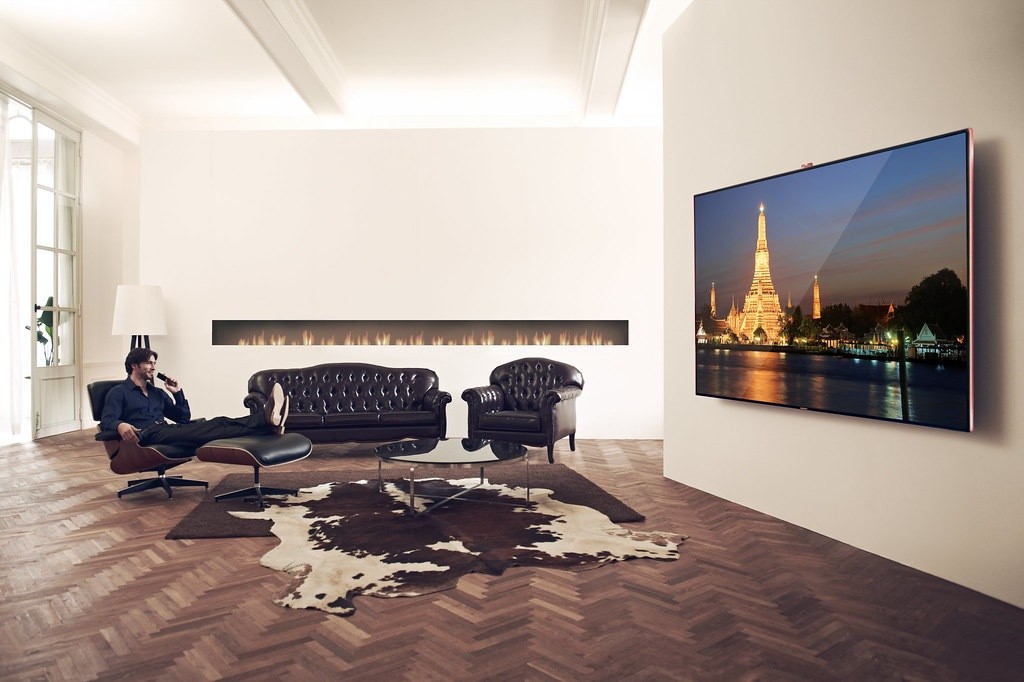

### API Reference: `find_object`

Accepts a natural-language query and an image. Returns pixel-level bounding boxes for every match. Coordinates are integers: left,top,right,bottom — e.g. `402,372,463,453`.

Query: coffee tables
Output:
373,437,529,519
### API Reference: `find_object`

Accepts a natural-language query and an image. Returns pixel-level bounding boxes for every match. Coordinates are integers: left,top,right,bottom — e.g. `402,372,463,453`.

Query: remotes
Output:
157,373,177,387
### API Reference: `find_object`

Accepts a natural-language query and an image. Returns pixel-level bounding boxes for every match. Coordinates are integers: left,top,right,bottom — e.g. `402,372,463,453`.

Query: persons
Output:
97,347,289,446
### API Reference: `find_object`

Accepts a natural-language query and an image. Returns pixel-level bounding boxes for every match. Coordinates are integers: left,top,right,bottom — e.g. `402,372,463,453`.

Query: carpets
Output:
228,477,686,616
165,462,688,539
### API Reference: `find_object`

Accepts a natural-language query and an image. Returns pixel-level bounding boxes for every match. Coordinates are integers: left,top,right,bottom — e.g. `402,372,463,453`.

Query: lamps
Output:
112,286,166,385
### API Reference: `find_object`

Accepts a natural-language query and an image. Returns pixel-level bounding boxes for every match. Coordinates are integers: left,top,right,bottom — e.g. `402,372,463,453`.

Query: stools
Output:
195,433,312,507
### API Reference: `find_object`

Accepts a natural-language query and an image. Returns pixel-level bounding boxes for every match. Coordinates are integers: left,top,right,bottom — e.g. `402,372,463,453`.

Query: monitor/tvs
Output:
693,127,977,433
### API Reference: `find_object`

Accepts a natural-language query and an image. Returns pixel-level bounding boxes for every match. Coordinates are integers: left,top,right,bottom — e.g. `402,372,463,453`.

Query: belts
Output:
141,420,165,436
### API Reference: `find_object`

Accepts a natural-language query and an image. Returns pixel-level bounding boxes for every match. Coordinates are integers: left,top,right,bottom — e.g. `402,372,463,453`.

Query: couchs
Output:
243,363,452,444
461,357,584,463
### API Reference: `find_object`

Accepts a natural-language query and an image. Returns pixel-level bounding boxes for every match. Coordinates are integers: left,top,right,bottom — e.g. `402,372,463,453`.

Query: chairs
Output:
86,380,209,501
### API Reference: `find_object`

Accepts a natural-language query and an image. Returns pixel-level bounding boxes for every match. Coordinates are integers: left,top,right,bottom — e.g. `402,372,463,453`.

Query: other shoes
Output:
272,395,289,435
265,383,284,426
